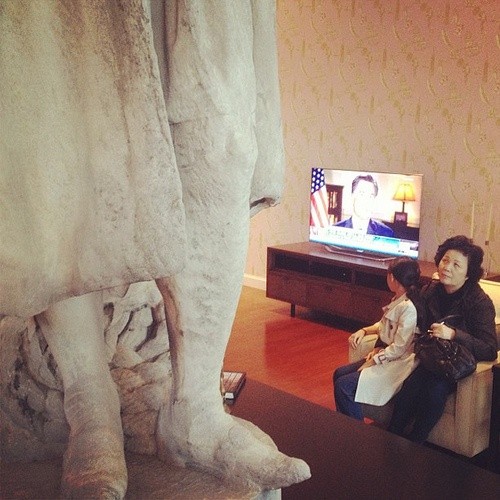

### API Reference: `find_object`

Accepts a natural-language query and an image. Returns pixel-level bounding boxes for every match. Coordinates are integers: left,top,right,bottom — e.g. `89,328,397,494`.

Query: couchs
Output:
349,272,500,457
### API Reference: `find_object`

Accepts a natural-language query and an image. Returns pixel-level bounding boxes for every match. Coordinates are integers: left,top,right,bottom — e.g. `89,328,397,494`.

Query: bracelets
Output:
361,328,367,336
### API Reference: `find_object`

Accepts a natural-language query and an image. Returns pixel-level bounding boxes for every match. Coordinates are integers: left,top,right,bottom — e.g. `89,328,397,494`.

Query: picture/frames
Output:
394,212,407,226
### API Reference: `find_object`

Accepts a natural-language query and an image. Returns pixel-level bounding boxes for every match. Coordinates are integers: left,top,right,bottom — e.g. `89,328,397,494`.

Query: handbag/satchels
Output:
417,313,476,384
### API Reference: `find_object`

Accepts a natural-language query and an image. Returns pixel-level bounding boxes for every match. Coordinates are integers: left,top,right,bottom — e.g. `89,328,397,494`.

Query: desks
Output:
227,379,500,500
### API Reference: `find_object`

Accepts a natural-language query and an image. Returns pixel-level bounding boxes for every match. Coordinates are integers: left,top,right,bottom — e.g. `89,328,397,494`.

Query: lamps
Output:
392,183,416,212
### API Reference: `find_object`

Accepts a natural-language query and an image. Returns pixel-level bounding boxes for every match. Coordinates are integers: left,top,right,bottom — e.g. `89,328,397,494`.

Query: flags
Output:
310,167,331,226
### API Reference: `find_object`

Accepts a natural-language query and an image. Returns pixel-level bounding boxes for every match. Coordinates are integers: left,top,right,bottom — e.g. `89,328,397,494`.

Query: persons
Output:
0,0,311,500
347,234,498,448
333,256,421,424
331,175,395,239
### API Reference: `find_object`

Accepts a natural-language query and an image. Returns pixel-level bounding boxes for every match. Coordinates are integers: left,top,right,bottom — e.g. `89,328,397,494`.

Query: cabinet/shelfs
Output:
266,241,437,324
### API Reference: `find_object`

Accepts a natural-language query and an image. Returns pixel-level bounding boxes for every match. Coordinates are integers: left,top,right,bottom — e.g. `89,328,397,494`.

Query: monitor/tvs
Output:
309,167,424,262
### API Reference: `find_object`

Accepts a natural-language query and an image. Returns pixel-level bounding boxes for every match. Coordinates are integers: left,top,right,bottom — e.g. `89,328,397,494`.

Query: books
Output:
220,370,247,400
